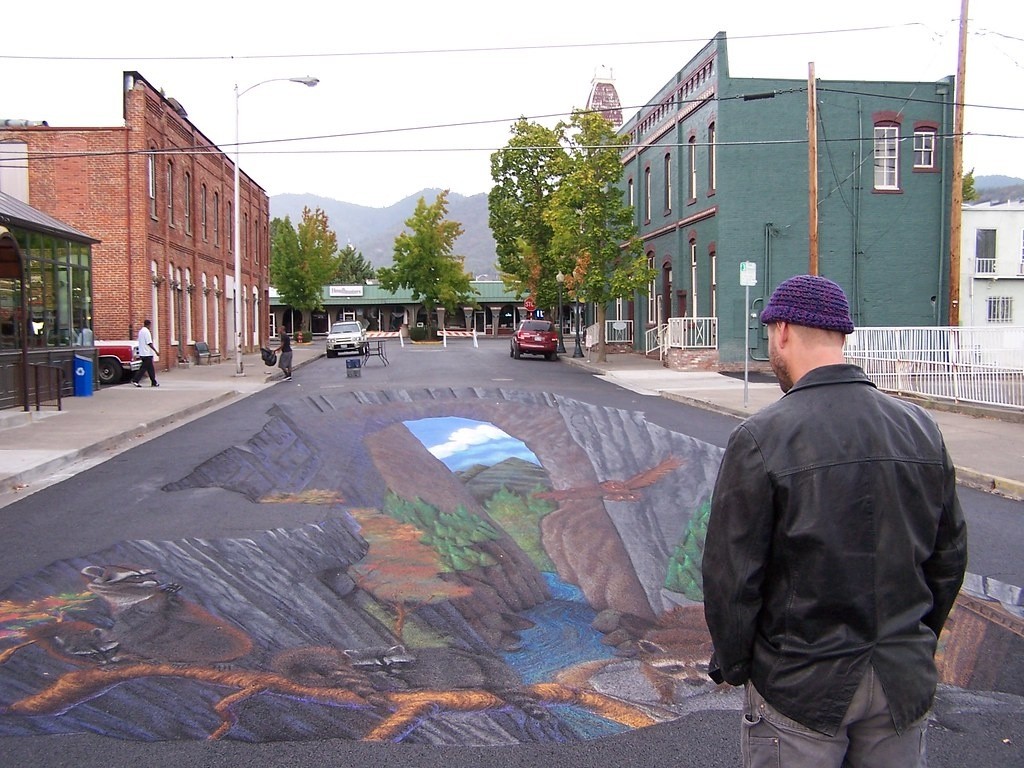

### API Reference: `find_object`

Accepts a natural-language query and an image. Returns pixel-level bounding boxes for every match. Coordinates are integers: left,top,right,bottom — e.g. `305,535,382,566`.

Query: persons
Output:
133,320,160,387
77,324,93,346
274,326,293,381
701,274,967,768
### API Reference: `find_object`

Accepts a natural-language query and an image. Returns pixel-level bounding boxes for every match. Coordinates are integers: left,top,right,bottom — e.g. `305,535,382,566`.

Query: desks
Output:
358,339,390,369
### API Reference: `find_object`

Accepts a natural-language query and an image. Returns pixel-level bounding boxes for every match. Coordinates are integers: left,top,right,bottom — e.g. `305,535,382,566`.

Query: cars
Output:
36,324,143,386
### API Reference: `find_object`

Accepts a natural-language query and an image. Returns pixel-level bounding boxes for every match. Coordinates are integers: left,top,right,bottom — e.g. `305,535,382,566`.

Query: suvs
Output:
510,321,558,362
326,321,368,357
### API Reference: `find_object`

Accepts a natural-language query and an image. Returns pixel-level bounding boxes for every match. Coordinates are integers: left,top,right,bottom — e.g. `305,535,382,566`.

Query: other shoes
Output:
151,383,160,387
131,381,141,387
282,376,292,382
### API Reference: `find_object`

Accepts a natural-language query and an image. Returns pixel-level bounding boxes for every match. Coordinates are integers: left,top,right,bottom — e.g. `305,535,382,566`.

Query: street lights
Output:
572,266,585,358
233,74,319,377
555,270,566,353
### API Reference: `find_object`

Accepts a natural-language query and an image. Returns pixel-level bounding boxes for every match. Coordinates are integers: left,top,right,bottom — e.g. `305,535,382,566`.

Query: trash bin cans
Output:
72,354,95,396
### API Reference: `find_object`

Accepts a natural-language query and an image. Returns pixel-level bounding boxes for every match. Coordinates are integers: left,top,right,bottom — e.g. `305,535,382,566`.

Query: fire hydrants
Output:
297,331,304,343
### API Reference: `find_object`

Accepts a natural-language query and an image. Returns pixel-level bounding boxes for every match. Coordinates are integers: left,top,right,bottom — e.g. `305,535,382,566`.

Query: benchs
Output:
195,341,222,366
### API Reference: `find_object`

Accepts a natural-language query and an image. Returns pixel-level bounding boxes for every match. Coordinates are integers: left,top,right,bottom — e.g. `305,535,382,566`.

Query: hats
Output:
760,275,855,333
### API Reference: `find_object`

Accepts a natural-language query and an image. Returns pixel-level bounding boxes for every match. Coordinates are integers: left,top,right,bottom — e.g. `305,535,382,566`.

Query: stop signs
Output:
525,298,536,311
